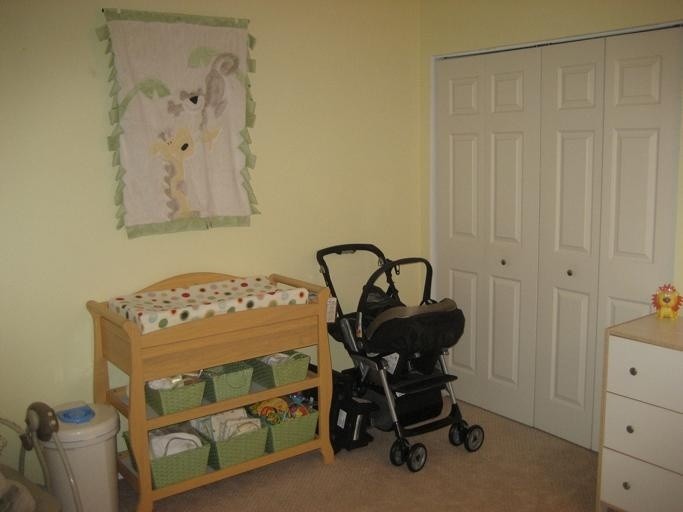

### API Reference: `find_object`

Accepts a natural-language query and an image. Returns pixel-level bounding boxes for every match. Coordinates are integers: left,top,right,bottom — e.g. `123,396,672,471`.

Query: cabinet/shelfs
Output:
533,27,683,452
433,48,534,432
84,271,336,511
594,311,683,512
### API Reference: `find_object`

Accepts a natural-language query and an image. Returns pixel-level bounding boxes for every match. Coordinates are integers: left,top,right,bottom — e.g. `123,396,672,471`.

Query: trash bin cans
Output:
38,399,120,512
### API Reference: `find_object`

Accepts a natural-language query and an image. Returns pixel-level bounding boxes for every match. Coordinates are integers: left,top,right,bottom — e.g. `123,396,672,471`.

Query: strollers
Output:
317,242,484,472
